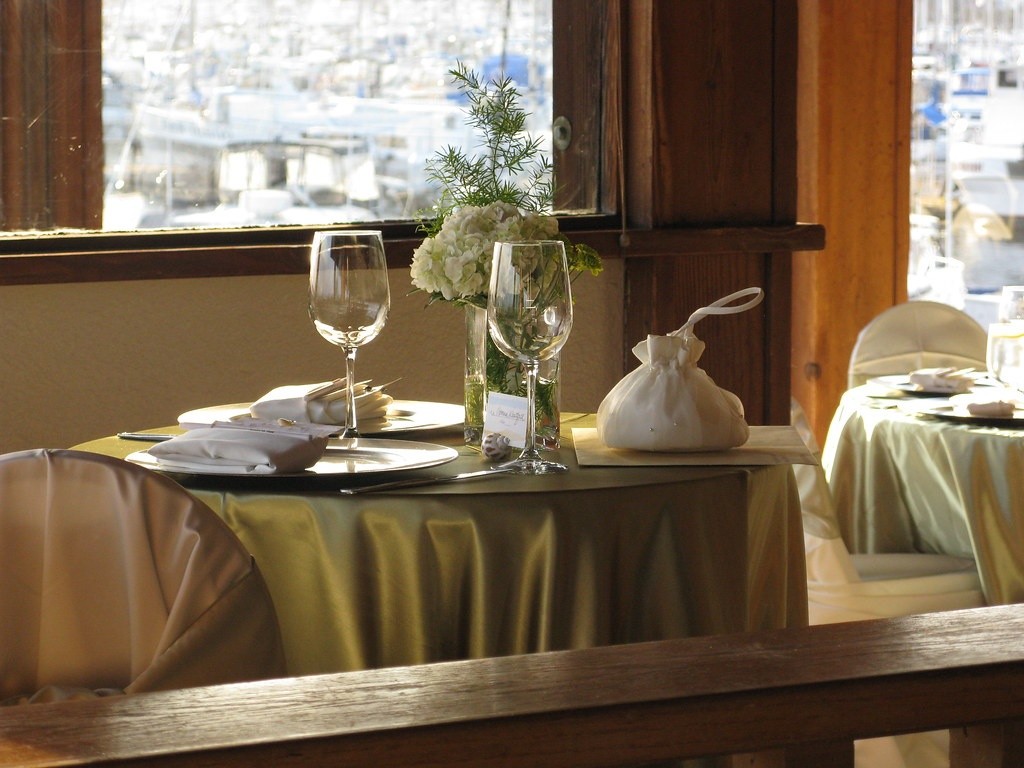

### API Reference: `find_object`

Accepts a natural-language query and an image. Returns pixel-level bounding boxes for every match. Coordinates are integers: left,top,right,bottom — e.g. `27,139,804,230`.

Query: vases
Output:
464,301,561,450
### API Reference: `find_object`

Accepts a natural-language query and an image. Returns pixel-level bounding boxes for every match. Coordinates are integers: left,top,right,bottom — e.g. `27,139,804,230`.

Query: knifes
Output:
340,468,519,496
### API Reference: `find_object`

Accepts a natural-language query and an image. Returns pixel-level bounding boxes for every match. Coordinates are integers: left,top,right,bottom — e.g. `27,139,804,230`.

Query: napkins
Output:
149,427,328,475
948,393,1016,417
252,381,394,426
909,366,978,392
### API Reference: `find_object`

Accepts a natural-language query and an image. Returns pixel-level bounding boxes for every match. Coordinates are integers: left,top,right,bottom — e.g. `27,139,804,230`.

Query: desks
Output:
823,368,1024,767
68,412,811,676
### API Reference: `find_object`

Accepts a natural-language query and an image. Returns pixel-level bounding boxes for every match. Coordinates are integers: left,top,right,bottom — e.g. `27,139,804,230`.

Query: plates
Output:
123,437,459,478
177,399,466,435
922,405,1024,421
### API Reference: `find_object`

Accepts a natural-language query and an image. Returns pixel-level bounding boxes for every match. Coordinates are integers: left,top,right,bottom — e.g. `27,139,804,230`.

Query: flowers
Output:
405,58,604,438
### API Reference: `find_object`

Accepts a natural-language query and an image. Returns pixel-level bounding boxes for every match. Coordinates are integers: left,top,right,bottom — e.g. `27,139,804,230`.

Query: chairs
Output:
789,395,984,768
0,449,286,708
846,301,988,388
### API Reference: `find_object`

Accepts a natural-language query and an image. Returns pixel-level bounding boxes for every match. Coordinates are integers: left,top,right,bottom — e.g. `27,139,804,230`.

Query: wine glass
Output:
487,239,574,476
307,230,391,438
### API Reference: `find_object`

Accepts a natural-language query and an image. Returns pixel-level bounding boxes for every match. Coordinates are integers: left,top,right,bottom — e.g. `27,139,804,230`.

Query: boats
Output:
907,23,1024,327
102,66,554,216
